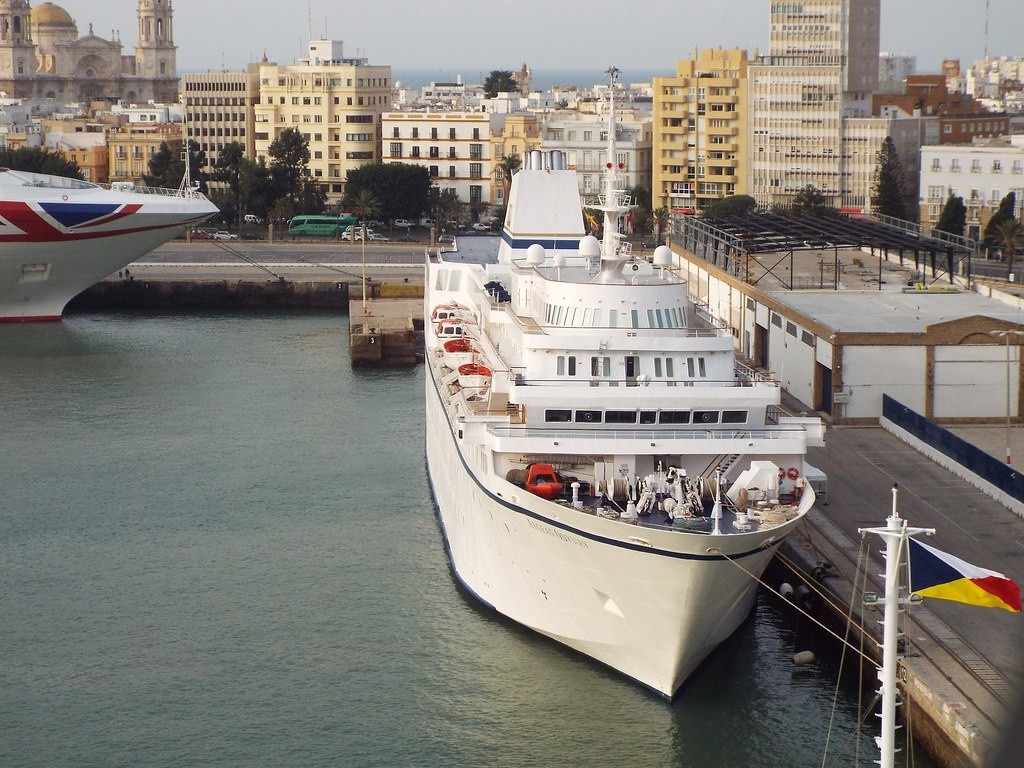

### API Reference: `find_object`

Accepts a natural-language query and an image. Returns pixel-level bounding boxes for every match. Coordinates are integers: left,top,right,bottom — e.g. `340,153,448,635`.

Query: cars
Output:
189,228,220,240
445,220,457,230
341,227,374,242
397,235,420,244
439,234,454,243
419,219,437,228
641,240,667,247
369,232,391,242
240,232,264,242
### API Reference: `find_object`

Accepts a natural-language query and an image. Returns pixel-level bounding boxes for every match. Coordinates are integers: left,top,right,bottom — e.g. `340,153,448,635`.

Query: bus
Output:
286,215,359,240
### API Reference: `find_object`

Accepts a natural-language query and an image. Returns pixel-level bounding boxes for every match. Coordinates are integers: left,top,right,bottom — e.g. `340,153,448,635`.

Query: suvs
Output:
394,219,416,231
472,223,491,231
212,230,238,241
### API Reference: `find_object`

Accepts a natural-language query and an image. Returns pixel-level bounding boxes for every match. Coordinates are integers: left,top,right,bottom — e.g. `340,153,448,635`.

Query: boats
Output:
420,66,832,706
0,161,220,322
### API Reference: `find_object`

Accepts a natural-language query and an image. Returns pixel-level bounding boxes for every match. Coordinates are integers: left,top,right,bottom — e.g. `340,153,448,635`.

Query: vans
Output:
244,215,260,224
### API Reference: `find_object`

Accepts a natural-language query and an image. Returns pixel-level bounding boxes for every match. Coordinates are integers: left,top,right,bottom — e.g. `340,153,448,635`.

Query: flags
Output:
905,536,1024,613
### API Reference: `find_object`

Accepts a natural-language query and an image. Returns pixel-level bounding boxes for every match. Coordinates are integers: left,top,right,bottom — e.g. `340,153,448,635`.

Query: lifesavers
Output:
779,467,786,479
787,467,799,480
